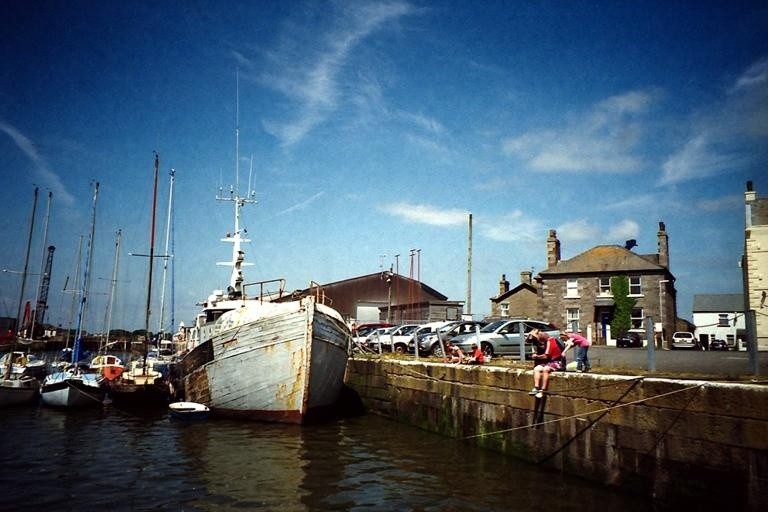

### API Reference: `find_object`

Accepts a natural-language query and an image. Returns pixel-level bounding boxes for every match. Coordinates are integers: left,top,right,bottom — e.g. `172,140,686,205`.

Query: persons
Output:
236,251,243,269
235,272,244,287
525,327,591,399
445,340,485,365
226,285,242,299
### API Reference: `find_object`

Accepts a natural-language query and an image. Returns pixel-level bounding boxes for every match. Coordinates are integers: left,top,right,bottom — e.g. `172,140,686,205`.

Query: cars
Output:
353,322,452,355
408,322,489,360
710,340,729,351
450,319,565,358
616,332,640,348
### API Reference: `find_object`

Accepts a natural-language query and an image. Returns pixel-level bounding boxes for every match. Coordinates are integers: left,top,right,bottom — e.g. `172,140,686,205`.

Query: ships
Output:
180,414,343,512
167,64,352,426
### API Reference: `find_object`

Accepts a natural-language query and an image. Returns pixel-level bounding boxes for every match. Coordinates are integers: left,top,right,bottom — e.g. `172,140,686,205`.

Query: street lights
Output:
394,247,422,320
659,279,669,350
381,263,393,322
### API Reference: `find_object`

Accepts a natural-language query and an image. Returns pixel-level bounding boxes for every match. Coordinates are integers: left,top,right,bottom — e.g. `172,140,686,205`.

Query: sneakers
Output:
535,390,543,399
529,390,538,395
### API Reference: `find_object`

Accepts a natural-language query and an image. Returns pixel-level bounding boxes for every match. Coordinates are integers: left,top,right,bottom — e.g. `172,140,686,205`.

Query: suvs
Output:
671,331,697,349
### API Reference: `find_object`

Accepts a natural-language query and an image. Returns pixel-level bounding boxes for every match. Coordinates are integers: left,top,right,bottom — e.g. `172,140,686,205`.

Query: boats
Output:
168,402,210,415
